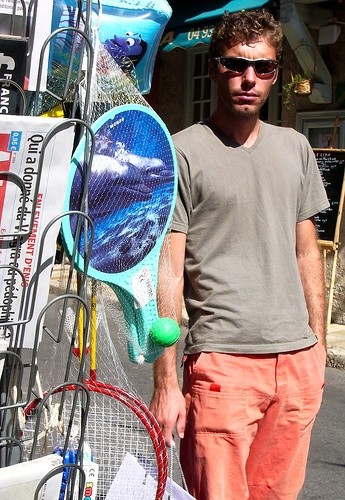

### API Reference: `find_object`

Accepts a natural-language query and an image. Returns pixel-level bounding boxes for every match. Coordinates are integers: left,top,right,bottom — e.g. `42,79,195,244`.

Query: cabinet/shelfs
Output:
0,0,95,500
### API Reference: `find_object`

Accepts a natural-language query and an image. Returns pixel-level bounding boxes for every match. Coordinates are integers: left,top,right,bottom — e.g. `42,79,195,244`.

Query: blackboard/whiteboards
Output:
312,148,345,246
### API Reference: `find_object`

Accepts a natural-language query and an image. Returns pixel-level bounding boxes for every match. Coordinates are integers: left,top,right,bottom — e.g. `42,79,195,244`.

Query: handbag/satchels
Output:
103,438,196,500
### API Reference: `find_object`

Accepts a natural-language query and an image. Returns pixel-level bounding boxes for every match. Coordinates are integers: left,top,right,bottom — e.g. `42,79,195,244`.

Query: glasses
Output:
214,56,278,78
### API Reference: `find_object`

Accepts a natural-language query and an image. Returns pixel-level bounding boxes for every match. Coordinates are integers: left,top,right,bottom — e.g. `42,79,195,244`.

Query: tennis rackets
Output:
62,103,178,364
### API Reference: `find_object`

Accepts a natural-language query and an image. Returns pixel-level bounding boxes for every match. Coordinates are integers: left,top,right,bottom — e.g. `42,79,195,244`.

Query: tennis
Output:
150,317,181,347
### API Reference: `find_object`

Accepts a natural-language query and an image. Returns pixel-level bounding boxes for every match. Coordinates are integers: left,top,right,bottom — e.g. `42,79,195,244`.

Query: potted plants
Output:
283,70,314,110
